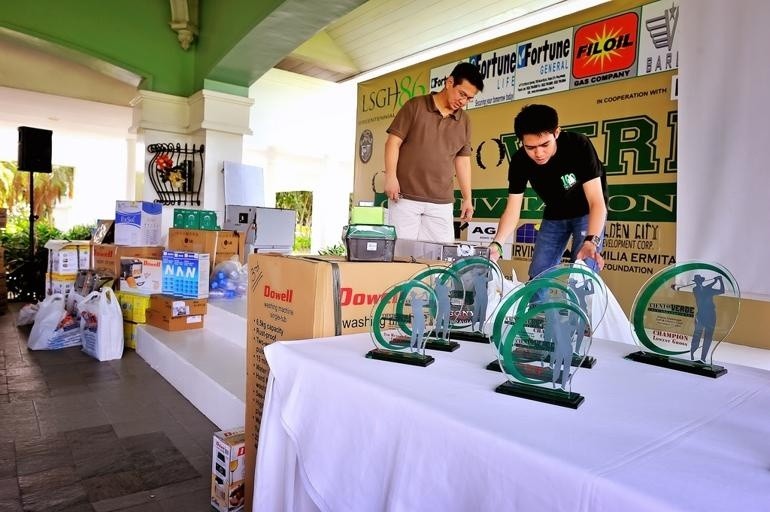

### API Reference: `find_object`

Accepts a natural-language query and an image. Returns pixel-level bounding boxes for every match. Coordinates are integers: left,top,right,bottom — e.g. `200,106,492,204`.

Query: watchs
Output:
583,235,601,247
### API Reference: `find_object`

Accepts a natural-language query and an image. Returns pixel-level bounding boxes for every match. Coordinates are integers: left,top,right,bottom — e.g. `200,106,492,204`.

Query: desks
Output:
249,318,770,511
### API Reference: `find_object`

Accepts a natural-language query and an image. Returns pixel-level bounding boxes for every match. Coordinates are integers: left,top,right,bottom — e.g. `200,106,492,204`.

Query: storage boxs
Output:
42,200,245,350
209,426,246,512
345,224,396,263
244,243,293,265
223,204,297,247
246,250,452,511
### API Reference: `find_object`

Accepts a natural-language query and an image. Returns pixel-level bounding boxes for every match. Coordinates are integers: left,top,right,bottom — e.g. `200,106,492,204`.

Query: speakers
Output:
17,125,53,172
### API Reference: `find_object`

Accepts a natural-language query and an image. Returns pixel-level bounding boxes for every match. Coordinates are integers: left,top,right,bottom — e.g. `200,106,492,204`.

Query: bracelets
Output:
489,242,503,256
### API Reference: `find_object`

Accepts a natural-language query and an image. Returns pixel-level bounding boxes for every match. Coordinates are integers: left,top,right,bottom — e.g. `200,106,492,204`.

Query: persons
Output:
485,104,607,336
383,62,484,260
